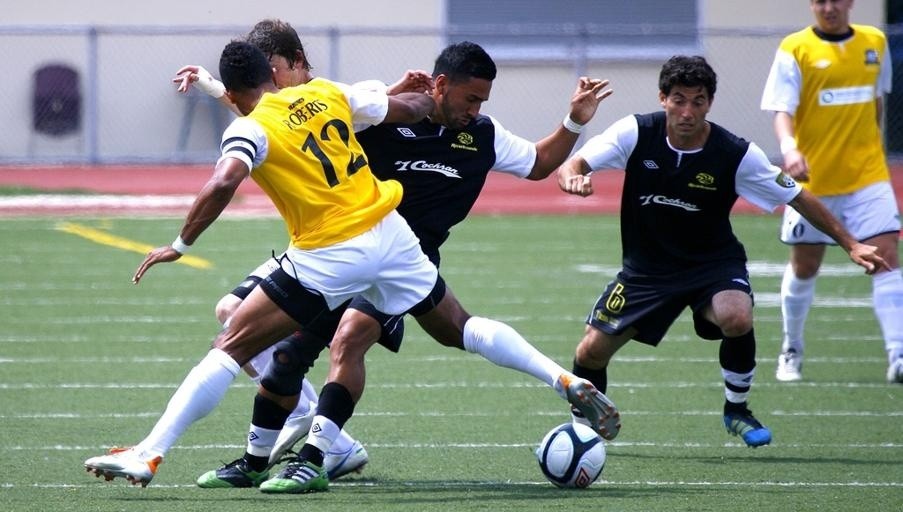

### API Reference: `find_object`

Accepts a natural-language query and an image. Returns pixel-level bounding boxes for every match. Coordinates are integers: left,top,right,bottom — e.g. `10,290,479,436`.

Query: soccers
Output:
538,423,606,488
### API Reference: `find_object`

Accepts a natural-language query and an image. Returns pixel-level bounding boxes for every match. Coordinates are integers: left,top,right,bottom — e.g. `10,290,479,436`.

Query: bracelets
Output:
170,235,191,258
562,114,584,136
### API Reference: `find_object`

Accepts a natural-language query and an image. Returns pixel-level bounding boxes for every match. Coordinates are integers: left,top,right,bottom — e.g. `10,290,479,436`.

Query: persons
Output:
169,16,371,483
551,54,895,452
192,38,620,495
757,1,903,385
81,39,622,491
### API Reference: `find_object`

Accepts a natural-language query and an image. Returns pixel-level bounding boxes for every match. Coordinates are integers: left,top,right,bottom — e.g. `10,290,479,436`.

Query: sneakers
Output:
83,448,161,488
887,355,902,383
196,403,368,494
554,370,620,440
777,342,804,381
723,405,770,448
